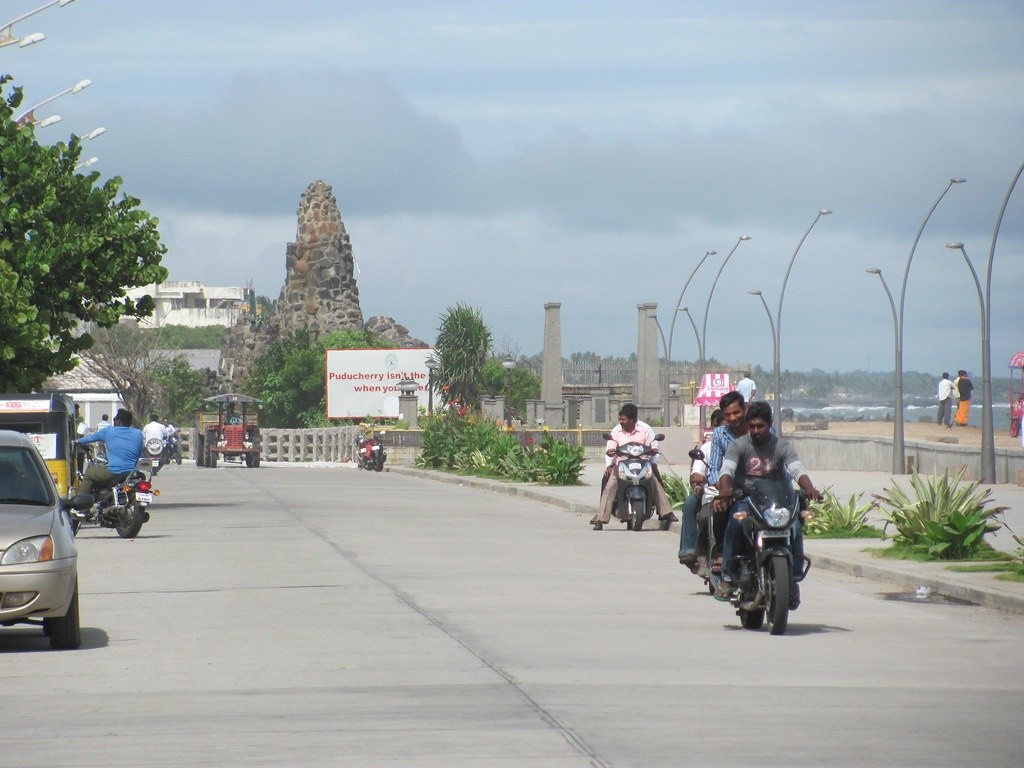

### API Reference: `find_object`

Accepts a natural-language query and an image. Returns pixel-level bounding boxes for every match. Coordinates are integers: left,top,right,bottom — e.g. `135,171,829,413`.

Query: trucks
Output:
195,392,265,469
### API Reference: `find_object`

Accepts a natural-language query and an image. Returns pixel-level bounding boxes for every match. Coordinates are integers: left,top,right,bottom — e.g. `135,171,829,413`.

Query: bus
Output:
0,391,86,498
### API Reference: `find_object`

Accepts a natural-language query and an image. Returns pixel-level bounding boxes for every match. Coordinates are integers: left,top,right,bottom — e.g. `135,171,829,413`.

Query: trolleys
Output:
1006,351,1024,438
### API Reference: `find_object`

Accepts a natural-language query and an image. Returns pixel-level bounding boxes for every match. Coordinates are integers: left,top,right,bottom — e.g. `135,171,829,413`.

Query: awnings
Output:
692,372,735,406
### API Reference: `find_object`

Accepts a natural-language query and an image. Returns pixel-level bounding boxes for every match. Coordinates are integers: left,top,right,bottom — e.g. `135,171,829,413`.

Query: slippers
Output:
712,582,734,603
788,582,801,611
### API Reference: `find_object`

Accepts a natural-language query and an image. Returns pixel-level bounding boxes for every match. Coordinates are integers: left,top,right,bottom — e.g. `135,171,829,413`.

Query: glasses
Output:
618,416,627,423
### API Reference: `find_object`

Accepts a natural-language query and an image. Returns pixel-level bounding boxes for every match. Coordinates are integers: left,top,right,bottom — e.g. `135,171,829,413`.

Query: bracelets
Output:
694,485,702,494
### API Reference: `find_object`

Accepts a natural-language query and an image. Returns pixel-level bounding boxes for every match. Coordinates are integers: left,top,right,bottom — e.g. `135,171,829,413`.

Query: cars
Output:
0,427,97,649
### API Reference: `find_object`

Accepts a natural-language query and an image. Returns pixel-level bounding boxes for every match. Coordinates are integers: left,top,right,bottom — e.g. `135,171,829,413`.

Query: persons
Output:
953,370,973,426
736,372,757,409
69,408,143,518
142,414,178,465
938,373,955,428
714,402,823,600
678,391,776,573
74,404,111,480
222,409,242,424
590,403,679,530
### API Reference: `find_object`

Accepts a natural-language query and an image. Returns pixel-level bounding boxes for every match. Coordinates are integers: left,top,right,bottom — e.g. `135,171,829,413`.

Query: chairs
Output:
0,461,23,500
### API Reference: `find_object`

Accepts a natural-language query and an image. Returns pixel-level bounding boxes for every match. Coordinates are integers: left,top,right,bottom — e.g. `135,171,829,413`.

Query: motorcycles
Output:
351,425,388,472
600,432,666,531
680,448,735,596
67,439,162,540
143,427,184,476
713,475,826,636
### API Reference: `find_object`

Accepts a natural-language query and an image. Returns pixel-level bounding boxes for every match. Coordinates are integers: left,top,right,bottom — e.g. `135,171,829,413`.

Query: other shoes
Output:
593,521,602,531
70,507,85,518
679,555,695,565
658,512,679,522
590,514,598,524
696,555,709,576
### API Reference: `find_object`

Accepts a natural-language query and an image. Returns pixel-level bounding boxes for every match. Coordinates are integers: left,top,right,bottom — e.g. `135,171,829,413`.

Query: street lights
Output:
864,175,969,475
646,248,718,427
944,160,1024,484
746,207,836,438
676,234,753,429
